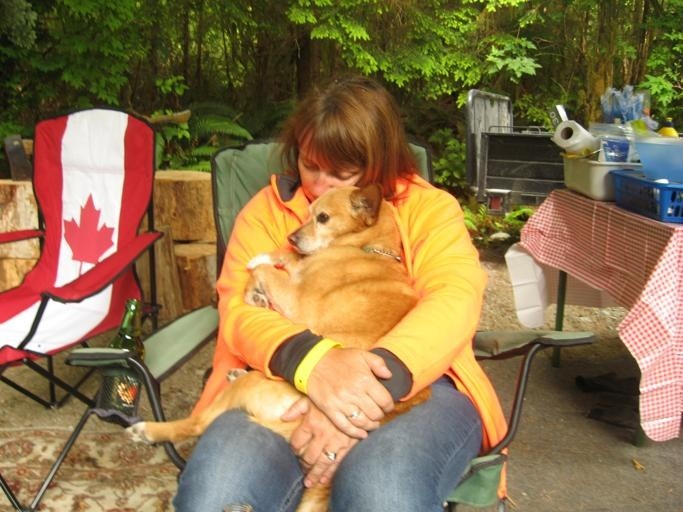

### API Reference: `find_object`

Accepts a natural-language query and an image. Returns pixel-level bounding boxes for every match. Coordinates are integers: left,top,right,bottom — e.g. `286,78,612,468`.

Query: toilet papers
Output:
552,120,596,156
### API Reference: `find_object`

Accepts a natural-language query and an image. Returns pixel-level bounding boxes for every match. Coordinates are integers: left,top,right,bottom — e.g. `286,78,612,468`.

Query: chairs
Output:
25,132,593,509
0,111,167,416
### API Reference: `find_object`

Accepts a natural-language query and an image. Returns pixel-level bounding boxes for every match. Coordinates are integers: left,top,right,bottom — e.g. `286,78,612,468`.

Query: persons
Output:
172,70,510,512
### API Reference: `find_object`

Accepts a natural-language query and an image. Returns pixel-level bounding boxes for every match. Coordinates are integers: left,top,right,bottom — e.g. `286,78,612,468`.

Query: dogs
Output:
122,171,434,512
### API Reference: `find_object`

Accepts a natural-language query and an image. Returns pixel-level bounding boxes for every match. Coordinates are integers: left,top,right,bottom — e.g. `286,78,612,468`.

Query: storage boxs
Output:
562,136,682,202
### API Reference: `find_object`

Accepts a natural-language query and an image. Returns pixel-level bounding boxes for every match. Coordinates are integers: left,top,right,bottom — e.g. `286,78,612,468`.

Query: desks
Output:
550,188,683,369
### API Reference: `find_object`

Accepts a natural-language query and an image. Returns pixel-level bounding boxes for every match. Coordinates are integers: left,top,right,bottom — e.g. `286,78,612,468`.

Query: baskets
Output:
608,168,683,227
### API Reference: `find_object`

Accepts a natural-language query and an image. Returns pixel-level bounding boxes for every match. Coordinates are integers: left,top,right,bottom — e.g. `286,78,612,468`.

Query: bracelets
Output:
293,337,344,396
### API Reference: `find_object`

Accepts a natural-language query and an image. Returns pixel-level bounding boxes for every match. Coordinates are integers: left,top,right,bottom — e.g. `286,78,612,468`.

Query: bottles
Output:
93,299,145,418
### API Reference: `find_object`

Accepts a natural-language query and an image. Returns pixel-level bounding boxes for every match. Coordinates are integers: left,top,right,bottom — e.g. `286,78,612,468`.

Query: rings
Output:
324,450,338,461
347,409,361,419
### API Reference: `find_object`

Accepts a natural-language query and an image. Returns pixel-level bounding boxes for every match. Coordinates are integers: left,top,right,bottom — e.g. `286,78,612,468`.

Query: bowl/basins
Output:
636,136,683,184
